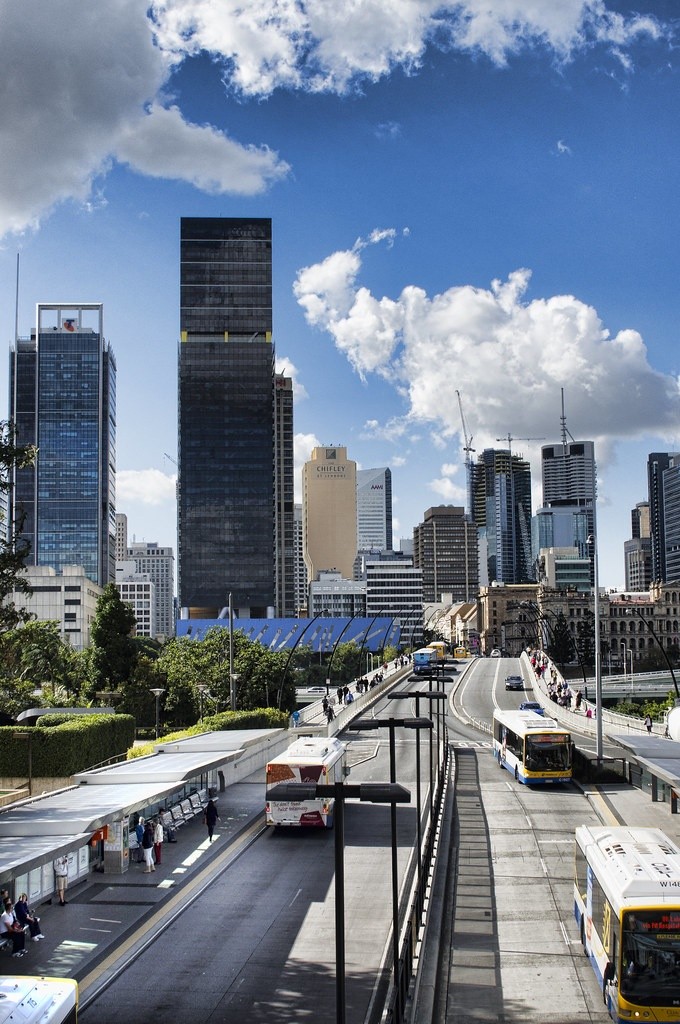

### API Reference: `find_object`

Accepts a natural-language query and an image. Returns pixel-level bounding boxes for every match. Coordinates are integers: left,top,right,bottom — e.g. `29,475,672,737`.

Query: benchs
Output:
0,902,41,952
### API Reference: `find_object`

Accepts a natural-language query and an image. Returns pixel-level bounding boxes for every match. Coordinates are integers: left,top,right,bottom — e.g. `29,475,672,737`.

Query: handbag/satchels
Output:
11,916,21,930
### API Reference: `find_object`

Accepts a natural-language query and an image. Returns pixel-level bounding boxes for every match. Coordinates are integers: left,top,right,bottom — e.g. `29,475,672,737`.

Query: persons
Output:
547,663,582,709
643,714,652,734
357,673,383,693
337,684,353,705
0,889,45,958
53,856,68,906
293,710,300,727
323,696,336,722
393,653,411,669
526,646,548,678
383,662,388,671
204,800,221,842
584,703,592,717
134,813,175,873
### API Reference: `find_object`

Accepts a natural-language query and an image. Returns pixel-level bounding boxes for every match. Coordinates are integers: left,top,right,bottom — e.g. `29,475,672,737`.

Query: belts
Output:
57,875,67,877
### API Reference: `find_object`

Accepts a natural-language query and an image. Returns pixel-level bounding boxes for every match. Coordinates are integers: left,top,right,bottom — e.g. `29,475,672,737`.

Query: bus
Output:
491,708,577,787
263,737,351,831
426,641,448,659
412,648,438,676
0,975,78,1024
570,825,680,1024
454,647,467,658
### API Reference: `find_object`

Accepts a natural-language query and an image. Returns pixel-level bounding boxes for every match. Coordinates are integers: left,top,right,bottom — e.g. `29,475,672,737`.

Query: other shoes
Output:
142,870,151,873
151,869,155,872
154,862,161,865
137,859,147,864
32,936,40,942
168,839,178,843
36,933,44,939
59,899,68,906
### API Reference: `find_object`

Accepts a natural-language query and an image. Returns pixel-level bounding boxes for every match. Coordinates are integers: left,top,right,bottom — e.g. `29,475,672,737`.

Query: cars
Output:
519,702,545,716
491,649,501,658
308,687,327,694
501,651,511,658
505,675,525,691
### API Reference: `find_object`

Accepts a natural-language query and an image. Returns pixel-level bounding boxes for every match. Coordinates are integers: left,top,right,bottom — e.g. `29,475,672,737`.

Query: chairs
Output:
188,794,209,809
169,805,195,822
129,831,141,862
162,811,186,827
178,799,203,815
197,788,220,803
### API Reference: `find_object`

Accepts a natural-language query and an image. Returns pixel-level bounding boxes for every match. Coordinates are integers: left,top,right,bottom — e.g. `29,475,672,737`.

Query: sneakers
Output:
12,952,24,958
19,949,29,954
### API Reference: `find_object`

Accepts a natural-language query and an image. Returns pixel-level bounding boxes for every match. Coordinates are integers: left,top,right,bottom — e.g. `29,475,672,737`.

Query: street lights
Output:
357,603,394,677
195,684,208,725
420,666,458,787
387,691,447,885
626,649,634,692
545,608,588,700
624,608,680,701
428,658,459,766
514,599,565,676
277,608,329,712
349,715,434,1024
586,534,603,775
150,688,167,740
380,601,453,663
325,606,364,696
13,734,34,800
405,674,455,826
620,643,627,683
229,673,242,711
266,781,412,1024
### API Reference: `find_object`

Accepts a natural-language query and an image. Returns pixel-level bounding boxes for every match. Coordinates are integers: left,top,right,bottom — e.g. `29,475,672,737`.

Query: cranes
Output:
455,389,478,458
495,431,546,455
